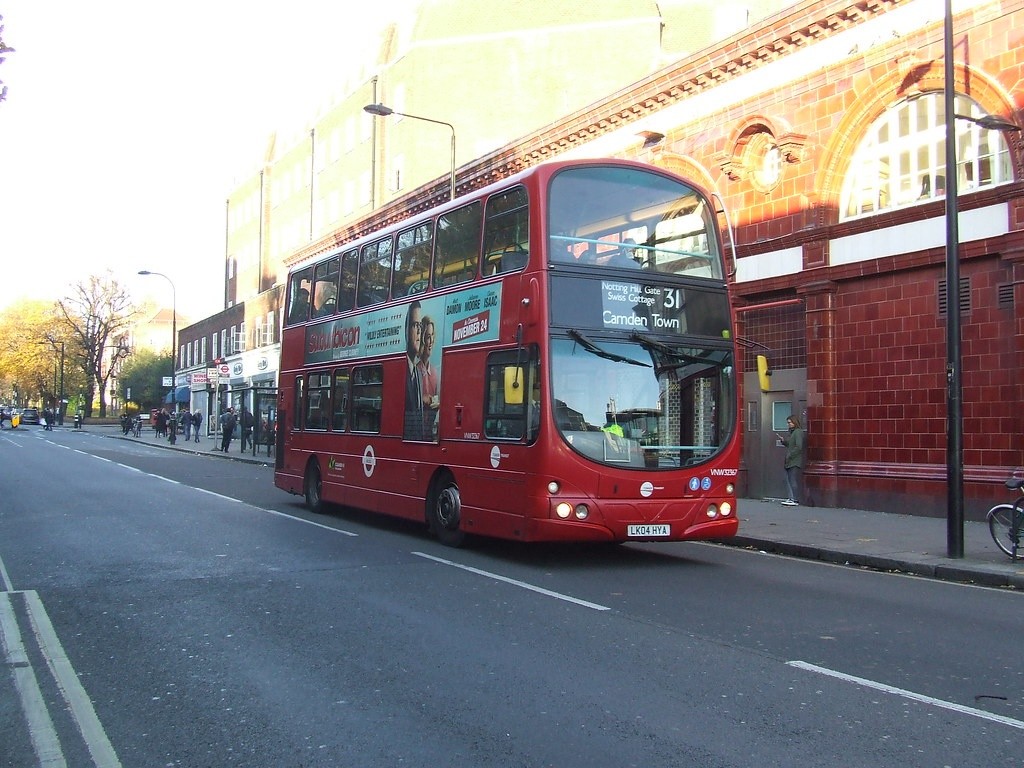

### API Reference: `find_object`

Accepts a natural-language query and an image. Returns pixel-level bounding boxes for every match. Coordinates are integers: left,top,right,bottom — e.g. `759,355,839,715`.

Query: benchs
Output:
255,429,275,457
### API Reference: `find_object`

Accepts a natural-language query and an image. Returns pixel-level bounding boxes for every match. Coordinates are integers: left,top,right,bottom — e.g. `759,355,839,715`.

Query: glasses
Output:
410,322,422,329
787,422,791,423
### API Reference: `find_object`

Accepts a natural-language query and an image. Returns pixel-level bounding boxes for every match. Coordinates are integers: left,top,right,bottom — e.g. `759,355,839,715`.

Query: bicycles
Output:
987,468,1024,564
132,419,143,438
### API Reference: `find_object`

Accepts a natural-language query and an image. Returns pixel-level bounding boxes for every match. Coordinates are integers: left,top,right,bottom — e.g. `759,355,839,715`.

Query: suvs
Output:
20,408,39,425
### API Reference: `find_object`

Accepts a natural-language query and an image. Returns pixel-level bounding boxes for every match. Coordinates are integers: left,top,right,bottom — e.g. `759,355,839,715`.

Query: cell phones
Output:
777,434,781,438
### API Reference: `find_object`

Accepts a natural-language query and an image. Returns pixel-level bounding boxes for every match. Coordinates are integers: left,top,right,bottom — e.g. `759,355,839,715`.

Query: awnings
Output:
166,387,190,404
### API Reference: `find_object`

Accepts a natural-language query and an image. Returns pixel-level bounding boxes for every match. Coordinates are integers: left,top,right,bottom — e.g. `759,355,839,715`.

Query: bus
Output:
272,156,772,549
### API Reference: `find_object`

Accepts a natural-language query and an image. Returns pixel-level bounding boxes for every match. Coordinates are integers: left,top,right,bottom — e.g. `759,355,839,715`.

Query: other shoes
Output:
225,450,229,453
780,499,799,506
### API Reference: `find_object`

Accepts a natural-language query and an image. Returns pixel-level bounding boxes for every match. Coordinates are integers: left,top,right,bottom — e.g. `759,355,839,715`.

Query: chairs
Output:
316,241,597,319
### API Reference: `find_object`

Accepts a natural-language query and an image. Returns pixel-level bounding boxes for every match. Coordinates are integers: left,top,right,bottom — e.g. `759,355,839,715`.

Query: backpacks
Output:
245,412,254,428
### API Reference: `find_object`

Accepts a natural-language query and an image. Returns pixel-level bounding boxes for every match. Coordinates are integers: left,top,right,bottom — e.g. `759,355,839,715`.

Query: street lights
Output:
363,104,456,202
138,270,176,445
38,341,64,425
944,0,1024,561
211,357,221,451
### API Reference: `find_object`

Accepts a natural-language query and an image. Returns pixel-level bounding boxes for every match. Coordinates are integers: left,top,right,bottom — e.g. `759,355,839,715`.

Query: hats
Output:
227,408,231,412
195,409,202,414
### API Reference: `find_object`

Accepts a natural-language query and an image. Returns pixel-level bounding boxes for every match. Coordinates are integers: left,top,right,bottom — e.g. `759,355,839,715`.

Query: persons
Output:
779,415,804,506
118,407,204,443
606,238,643,269
78,406,85,429
507,381,568,440
0,405,57,430
404,300,439,441
288,262,411,324
218,405,269,454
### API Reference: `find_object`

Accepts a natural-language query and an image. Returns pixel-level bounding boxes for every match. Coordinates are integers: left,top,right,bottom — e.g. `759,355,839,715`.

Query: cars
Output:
0,404,22,424
